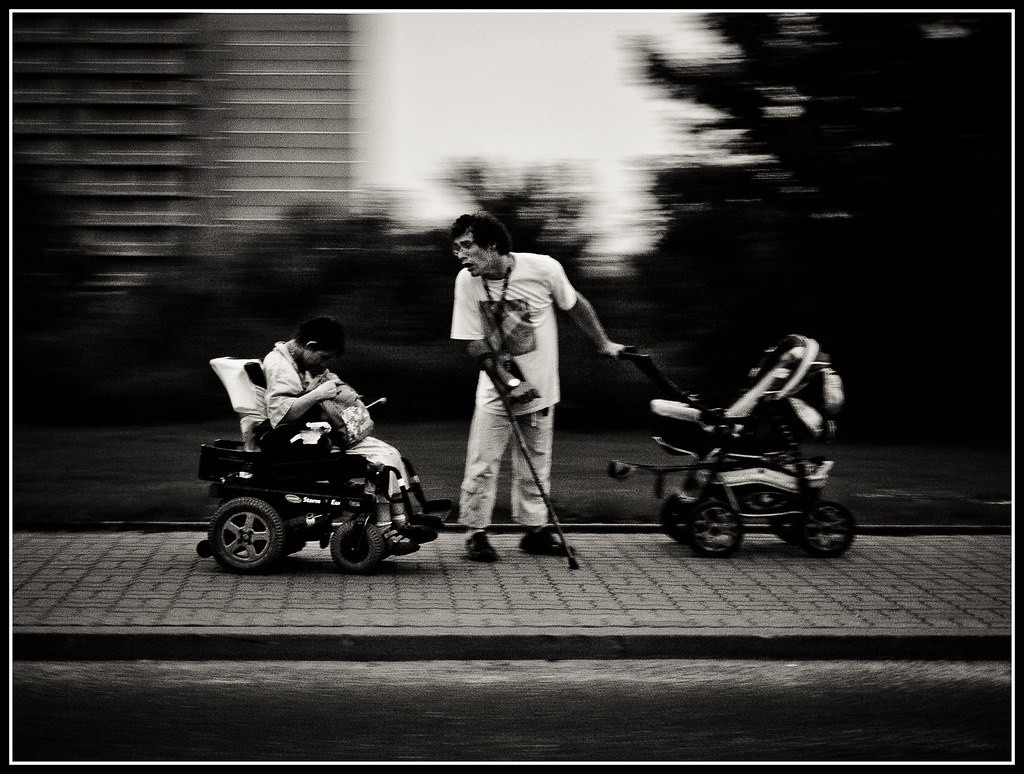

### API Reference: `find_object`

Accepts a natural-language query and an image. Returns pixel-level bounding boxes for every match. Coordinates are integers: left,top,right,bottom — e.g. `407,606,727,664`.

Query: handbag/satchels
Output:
306,373,375,447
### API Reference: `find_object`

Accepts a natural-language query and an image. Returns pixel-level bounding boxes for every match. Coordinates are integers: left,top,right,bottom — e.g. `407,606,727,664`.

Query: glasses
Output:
454,240,477,253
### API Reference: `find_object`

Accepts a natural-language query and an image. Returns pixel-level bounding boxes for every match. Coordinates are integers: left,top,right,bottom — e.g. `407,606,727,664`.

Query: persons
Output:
448,214,626,564
262,316,439,557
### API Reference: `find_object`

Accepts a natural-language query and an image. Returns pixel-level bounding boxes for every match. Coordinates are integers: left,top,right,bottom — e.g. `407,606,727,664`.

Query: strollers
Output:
601,332,856,560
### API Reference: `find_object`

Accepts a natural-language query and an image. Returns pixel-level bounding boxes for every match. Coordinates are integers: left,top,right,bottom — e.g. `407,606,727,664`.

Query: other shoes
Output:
375,524,417,553
518,527,573,556
395,516,438,537
465,529,498,561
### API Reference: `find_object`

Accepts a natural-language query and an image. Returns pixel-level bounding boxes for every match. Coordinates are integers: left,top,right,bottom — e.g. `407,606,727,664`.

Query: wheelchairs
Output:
195,356,458,571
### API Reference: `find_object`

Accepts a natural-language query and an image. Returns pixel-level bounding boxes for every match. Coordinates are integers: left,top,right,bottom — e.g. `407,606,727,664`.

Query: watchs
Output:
505,378,521,393
